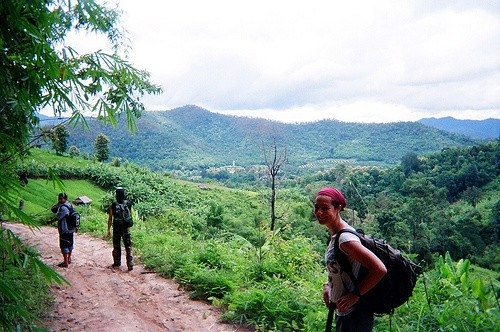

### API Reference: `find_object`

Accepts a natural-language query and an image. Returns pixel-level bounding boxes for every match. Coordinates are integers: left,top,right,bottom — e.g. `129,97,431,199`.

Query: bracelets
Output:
323,283,329,287
50,219,53,225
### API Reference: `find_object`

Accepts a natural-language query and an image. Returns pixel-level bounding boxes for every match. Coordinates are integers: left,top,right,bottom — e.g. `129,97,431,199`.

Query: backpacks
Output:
114,200,133,228
59,204,80,233
326,228,422,317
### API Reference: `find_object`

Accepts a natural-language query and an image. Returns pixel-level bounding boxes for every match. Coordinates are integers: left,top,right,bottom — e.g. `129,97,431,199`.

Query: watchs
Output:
352,288,362,298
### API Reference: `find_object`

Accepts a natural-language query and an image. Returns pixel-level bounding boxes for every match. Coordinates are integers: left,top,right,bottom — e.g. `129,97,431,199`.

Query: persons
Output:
314,186,387,332
107,187,133,271
47,193,78,268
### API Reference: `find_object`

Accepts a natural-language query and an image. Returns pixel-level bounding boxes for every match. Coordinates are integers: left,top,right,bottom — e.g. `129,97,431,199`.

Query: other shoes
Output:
58,256,72,267
112,263,134,271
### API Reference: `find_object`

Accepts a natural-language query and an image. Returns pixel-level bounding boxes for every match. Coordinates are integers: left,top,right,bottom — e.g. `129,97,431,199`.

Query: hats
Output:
115,187,124,193
318,187,346,211
